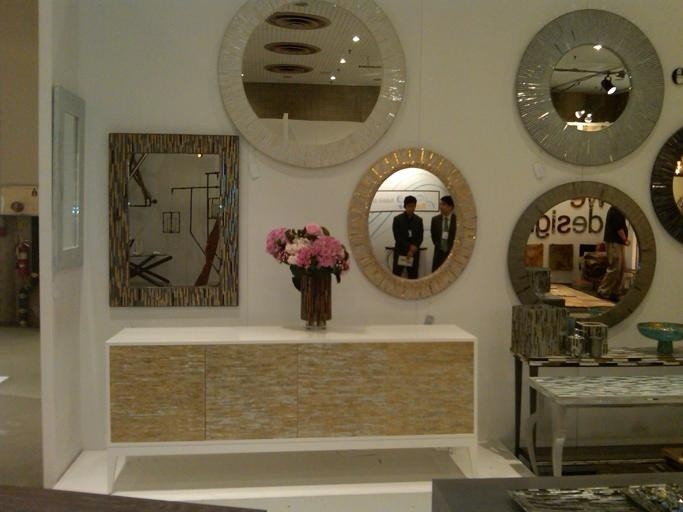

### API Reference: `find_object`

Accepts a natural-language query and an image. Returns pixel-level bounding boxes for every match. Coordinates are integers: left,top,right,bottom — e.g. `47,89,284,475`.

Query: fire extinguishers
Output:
15,235,33,273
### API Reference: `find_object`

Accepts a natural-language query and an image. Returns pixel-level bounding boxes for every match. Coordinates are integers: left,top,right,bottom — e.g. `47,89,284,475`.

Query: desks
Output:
0,480,270,511
379,244,429,279
125,249,175,289
504,349,682,473
427,467,683,511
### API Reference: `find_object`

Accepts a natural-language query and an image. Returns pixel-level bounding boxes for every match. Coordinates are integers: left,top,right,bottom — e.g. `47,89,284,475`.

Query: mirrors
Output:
506,180,659,333
644,125,683,247
212,0,410,172
107,132,241,309
512,6,670,167
344,145,483,303
50,84,86,273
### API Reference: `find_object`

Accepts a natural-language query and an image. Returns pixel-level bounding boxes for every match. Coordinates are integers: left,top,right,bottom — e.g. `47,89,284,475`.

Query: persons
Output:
392,195,424,279
430,196,456,272
596,207,631,303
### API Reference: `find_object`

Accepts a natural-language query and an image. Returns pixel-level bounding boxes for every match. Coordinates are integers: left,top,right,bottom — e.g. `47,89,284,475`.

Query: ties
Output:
441,218,451,252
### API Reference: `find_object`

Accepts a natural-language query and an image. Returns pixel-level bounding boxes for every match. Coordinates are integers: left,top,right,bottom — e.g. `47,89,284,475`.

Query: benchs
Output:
522,367,682,478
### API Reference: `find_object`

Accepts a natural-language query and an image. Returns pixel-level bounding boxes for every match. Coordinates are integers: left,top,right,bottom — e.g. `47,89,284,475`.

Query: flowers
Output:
261,221,350,291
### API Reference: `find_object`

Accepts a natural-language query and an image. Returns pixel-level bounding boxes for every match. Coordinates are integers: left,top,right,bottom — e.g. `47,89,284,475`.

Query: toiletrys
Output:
564,328,586,357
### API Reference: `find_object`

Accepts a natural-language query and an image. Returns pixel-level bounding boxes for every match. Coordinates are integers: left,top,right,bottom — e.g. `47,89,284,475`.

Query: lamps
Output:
596,67,620,99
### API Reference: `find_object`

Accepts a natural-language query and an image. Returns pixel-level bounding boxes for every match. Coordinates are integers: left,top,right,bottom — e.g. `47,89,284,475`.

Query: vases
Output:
295,270,335,329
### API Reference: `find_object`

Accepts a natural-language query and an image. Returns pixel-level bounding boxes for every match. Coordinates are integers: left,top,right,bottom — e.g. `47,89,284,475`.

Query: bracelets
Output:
623,239,627,243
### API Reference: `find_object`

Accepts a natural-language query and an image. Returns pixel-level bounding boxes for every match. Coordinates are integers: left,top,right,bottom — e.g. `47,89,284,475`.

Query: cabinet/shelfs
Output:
101,319,483,497
